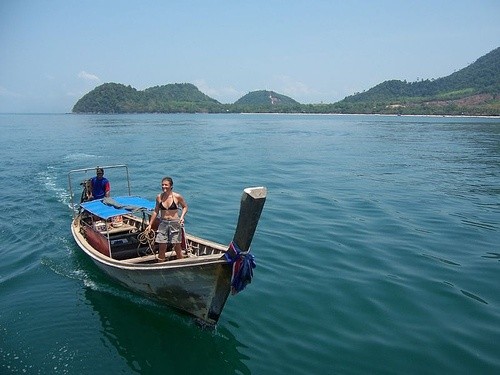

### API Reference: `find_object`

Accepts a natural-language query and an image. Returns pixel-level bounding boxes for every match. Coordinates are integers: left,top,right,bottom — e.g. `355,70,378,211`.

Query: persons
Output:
87,168,111,201
144,176,187,264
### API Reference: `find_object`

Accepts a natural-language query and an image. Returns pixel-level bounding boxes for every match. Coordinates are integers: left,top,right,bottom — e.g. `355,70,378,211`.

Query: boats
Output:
68,164,267,327
